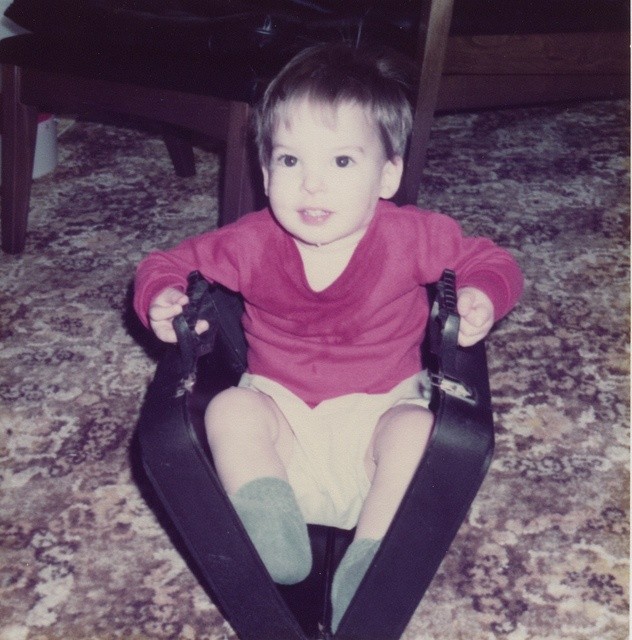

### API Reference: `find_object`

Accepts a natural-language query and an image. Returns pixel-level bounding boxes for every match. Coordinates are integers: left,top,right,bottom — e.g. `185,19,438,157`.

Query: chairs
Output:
2,2,445,256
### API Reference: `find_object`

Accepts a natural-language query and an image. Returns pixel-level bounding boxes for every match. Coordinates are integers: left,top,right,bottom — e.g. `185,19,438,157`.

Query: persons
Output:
133,43,524,634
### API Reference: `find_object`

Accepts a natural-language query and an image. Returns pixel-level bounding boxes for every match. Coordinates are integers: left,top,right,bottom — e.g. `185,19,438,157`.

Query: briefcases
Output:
136,269,496,640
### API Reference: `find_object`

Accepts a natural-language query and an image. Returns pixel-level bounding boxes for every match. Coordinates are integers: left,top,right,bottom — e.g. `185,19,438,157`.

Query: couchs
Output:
409,2,631,115
